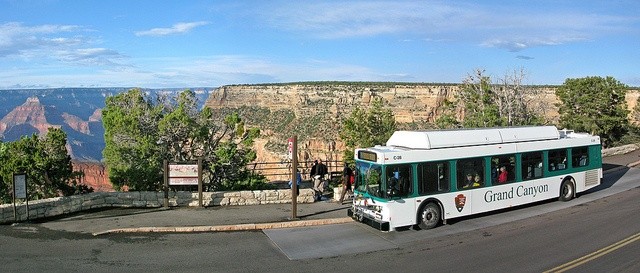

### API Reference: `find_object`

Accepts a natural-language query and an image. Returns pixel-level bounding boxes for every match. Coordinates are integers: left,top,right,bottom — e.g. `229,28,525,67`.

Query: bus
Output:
344,125,603,233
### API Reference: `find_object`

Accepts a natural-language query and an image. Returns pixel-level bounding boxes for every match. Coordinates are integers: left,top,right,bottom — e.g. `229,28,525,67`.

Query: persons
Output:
461,159,509,188
288,158,328,200
378,172,401,196
339,161,354,203
